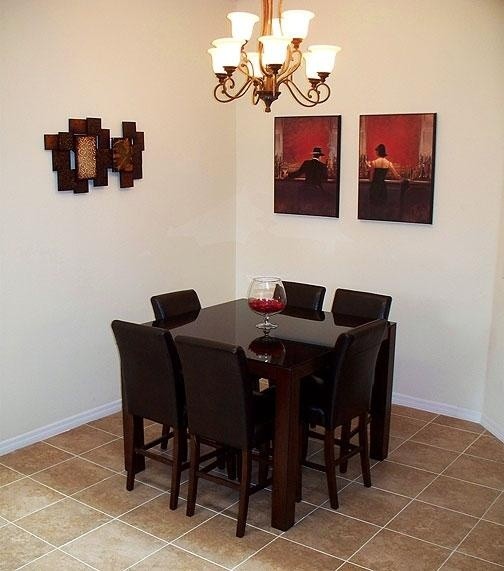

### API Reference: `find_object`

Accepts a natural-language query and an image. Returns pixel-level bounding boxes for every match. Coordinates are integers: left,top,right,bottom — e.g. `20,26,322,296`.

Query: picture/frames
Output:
274,115,341,218
358,113,437,225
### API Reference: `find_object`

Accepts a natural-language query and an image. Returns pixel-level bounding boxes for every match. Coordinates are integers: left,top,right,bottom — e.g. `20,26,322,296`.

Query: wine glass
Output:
247,277,286,330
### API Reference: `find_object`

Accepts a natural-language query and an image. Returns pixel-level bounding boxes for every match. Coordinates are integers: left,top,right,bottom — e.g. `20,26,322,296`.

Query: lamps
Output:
207,0,341,112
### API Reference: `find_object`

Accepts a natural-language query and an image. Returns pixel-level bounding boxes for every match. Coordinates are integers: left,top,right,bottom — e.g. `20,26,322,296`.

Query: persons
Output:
361,144,411,207
281,147,329,199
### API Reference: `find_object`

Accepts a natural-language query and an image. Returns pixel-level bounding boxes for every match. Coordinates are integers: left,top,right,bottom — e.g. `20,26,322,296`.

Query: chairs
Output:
111,281,397,538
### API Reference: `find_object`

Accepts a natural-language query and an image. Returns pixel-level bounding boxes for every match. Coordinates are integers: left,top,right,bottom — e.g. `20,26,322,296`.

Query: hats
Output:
310,148,325,156
